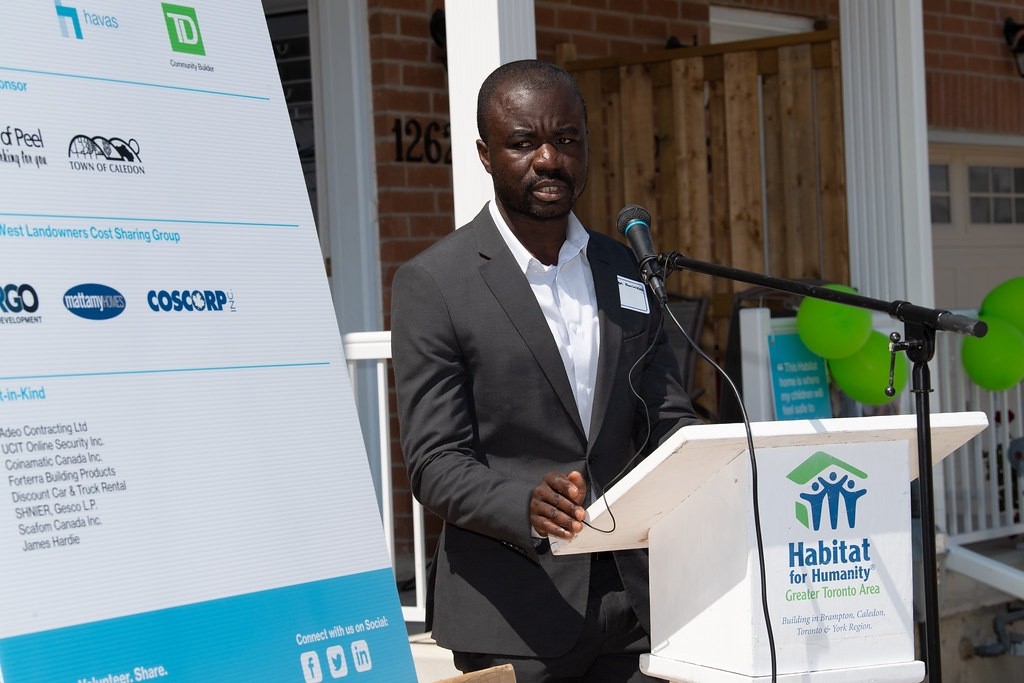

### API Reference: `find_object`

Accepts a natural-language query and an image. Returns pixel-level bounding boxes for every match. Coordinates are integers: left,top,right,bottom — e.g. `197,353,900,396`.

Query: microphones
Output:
617,204,669,305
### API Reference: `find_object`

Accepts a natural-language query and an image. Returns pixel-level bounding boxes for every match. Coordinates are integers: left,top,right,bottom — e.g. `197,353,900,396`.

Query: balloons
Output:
796,284,909,405
962,276,1024,391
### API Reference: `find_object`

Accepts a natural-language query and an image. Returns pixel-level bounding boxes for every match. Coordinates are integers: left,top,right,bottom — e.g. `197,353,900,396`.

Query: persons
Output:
391,59,706,683
